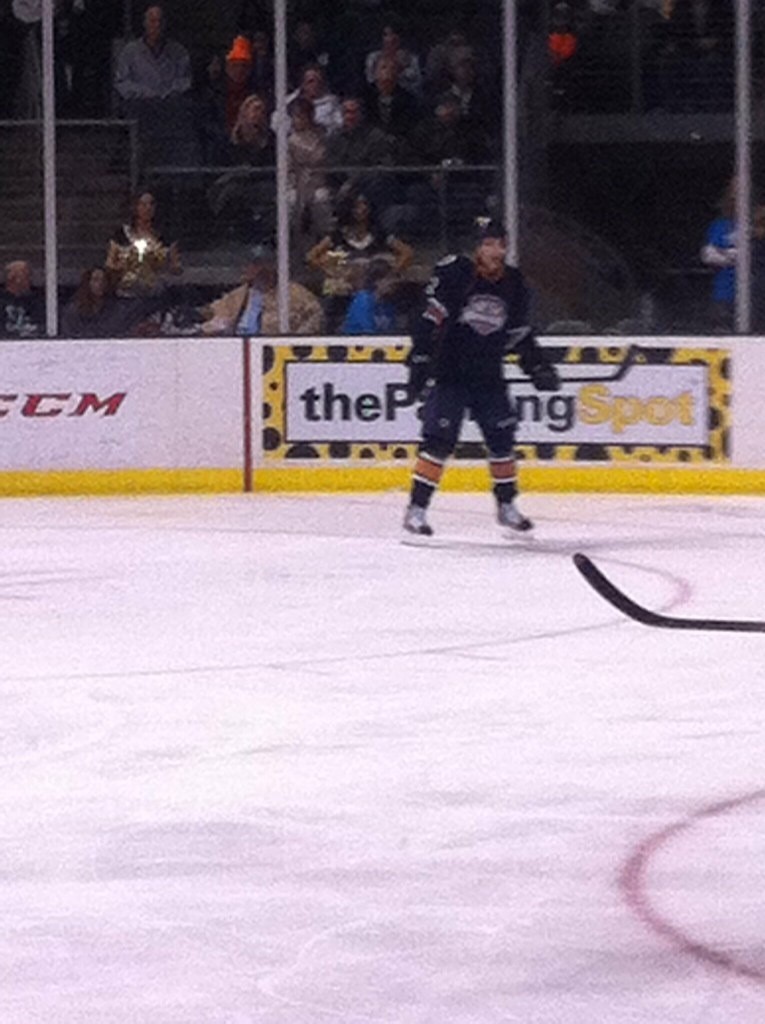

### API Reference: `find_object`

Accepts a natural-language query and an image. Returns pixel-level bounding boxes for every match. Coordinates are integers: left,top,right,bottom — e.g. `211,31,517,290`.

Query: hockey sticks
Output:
572,550,765,635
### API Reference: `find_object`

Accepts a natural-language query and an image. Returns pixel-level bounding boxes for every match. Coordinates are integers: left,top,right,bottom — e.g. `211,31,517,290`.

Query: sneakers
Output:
400,504,443,549
496,502,536,542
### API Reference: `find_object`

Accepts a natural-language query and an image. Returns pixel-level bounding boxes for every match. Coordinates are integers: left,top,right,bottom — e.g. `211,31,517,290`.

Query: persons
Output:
704,176,765,333
0,0,505,339
407,225,562,537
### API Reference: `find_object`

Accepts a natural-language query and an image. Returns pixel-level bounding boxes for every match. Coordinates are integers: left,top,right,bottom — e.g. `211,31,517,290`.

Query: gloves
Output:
532,365,561,392
410,362,435,392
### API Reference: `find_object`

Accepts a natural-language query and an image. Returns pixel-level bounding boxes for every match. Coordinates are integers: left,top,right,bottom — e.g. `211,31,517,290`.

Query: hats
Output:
464,217,506,252
